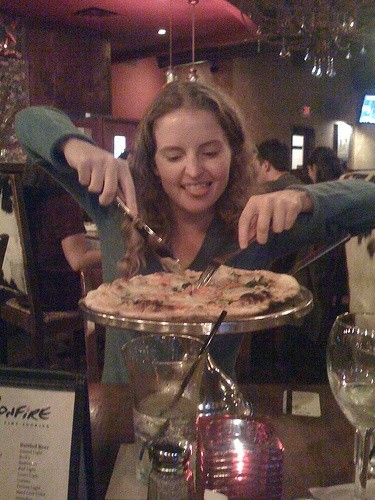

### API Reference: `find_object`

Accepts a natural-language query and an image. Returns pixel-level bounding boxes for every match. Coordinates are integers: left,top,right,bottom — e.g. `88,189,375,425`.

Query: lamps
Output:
239,0,375,80
167,0,200,82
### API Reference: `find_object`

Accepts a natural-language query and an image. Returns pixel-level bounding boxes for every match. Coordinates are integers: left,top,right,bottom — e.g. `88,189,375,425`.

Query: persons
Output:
301,144,341,184
15,80,375,384
29,181,102,370
252,139,305,192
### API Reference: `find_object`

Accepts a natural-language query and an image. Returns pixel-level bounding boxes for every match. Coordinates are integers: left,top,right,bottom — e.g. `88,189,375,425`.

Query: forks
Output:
194,226,271,288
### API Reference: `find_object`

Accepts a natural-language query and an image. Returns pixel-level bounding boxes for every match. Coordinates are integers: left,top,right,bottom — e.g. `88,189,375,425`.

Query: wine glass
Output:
325,310,375,500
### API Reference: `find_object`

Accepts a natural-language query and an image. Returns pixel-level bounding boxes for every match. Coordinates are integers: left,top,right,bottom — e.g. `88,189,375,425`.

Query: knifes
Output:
114,195,186,276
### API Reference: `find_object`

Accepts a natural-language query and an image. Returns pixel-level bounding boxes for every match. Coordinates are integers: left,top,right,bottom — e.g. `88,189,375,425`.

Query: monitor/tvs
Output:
358,93,375,125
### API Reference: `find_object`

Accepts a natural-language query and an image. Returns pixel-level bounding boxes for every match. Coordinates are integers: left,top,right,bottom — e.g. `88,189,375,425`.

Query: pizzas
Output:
85,264,299,319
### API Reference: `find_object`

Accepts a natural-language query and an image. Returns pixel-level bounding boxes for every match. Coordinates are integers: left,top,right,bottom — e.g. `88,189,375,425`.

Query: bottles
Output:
147,434,195,500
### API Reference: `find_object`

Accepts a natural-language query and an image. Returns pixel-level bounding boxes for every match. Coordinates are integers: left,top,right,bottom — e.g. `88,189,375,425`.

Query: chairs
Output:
0,171,85,372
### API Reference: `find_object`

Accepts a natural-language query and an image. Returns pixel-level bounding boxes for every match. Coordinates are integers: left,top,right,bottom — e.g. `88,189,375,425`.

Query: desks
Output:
83,380,358,500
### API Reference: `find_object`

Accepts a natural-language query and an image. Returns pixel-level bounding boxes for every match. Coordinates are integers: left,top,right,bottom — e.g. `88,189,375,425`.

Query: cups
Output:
120,333,209,485
195,415,285,500
